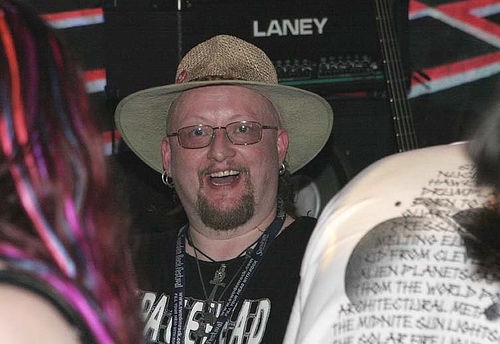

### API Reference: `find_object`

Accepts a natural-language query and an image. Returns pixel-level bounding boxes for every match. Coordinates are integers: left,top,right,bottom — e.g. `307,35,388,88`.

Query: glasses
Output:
165,120,280,150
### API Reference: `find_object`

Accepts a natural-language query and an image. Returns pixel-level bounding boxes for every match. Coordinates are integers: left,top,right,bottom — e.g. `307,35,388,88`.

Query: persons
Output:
0,1,148,344
113,34,335,343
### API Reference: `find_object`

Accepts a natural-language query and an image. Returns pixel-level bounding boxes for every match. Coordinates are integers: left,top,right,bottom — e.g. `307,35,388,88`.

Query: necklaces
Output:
187,225,263,340
183,225,264,304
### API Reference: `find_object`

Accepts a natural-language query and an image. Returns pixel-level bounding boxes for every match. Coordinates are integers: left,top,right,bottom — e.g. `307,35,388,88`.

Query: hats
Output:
114,33,332,174
